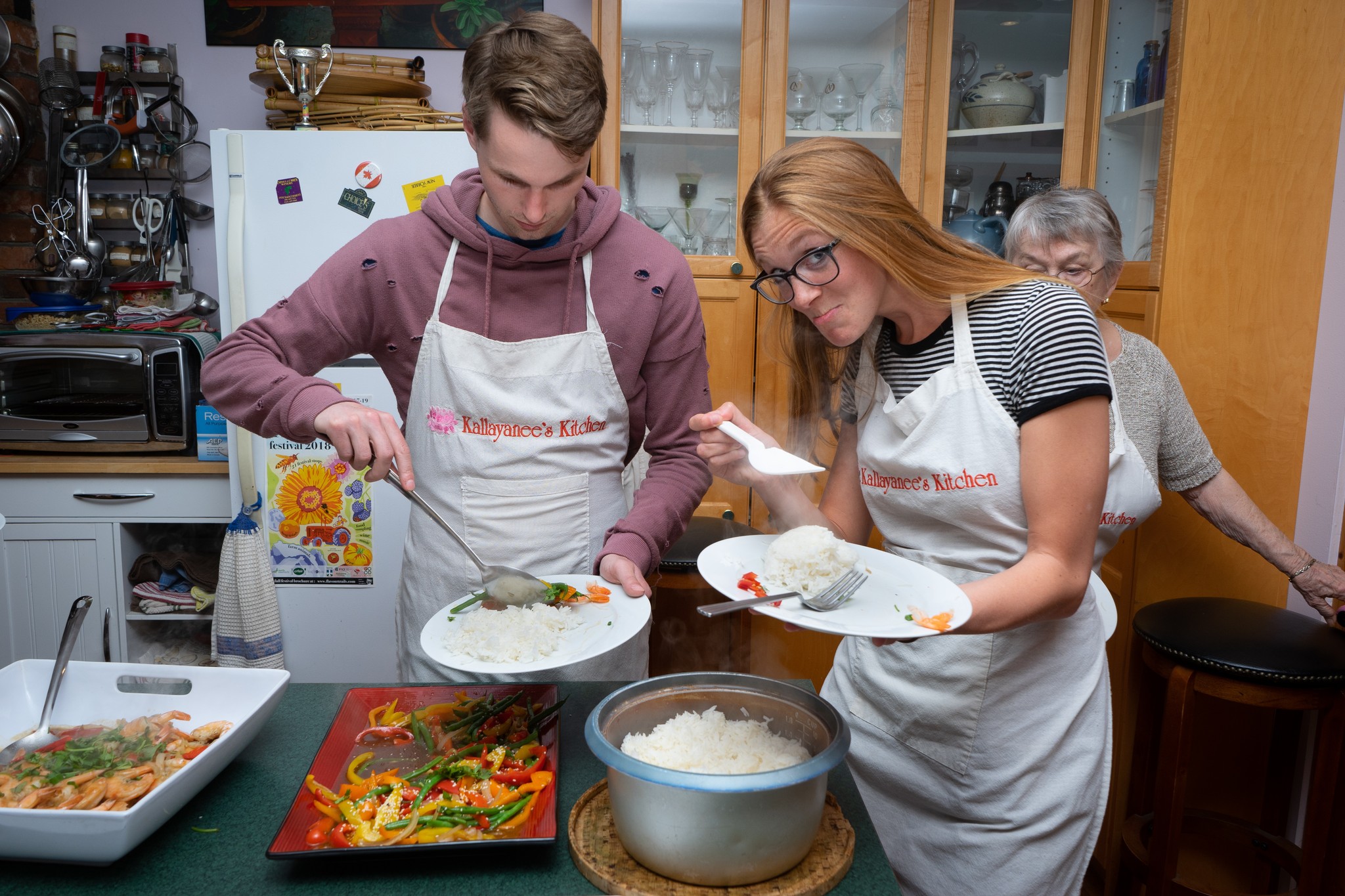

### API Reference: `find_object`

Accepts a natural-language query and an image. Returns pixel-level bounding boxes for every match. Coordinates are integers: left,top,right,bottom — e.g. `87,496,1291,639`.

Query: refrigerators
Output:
211,127,479,683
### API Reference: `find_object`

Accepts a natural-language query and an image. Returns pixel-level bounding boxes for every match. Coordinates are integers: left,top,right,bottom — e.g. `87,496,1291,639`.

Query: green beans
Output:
334,686,571,834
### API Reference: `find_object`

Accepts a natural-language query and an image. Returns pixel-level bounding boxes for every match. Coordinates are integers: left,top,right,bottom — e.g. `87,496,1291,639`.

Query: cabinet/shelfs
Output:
584,0,1345,670
0,475,236,662
46,72,192,291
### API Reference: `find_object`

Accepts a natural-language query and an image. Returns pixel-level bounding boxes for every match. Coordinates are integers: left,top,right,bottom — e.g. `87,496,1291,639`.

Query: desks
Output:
0,674,907,896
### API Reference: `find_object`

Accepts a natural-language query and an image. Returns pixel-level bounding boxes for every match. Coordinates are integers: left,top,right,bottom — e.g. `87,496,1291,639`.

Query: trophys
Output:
273,39,334,131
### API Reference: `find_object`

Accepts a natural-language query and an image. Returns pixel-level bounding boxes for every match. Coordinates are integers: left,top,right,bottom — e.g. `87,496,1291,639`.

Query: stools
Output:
645,513,765,674
1121,587,1345,896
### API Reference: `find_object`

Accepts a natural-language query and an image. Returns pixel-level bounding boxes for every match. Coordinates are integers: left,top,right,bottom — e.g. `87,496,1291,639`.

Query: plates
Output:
697,533,974,639
264,682,561,862
419,573,651,674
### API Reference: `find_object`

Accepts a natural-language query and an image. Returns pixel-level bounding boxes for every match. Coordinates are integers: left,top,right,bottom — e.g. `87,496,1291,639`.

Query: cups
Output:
1111,79,1136,115
947,33,979,131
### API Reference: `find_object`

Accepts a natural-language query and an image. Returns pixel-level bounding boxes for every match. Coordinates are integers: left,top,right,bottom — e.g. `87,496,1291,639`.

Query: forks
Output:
697,567,870,620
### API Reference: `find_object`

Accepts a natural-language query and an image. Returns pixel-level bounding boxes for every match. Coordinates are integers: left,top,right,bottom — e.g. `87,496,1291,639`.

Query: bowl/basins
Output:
0,658,290,864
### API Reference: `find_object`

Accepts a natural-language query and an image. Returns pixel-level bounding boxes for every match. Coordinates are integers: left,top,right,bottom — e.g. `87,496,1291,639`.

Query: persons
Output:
688,138,1112,896
200,13,715,682
1002,188,1345,629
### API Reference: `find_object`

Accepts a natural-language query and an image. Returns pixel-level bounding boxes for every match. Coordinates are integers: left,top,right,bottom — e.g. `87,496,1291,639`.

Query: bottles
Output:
960,63,1037,129
1015,171,1061,209
1134,28,1171,108
982,181,1015,220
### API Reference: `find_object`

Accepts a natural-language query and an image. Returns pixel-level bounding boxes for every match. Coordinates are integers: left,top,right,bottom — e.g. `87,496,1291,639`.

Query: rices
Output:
621,704,813,775
760,524,861,596
441,600,587,664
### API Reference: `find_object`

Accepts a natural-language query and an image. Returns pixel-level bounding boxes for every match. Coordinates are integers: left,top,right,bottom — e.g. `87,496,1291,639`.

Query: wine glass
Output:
785,61,903,133
620,38,740,129
633,183,737,257
945,165,974,190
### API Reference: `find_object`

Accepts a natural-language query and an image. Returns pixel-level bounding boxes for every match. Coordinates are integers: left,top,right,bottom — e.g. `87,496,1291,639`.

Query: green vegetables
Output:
447,585,590,621
0,721,187,799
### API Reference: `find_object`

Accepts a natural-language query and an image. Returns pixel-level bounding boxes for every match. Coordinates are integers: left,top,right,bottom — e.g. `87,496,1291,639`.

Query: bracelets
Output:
1288,559,1316,583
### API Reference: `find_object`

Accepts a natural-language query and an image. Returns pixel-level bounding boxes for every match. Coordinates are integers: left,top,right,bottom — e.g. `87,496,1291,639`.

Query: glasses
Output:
751,237,841,305
1025,261,1111,288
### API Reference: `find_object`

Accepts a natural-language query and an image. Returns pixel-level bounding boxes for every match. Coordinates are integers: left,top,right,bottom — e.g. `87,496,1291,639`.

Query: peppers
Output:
738,572,782,606
537,580,611,603
305,692,554,849
10,725,208,762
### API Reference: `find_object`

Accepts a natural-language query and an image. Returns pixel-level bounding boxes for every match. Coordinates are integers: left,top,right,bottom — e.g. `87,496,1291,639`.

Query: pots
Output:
584,671,852,887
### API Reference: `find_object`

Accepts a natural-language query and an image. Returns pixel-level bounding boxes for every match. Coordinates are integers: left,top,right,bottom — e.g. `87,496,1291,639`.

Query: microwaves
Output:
0,326,202,454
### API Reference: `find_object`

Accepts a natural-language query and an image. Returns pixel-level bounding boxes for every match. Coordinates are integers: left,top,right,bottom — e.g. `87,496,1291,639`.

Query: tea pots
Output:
945,208,1009,257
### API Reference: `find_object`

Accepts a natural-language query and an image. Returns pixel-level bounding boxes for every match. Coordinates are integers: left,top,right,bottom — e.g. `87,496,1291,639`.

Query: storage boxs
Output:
193,401,229,464
